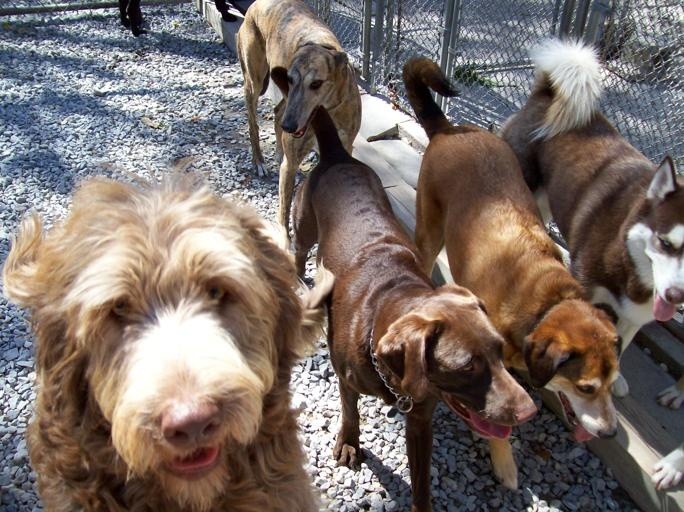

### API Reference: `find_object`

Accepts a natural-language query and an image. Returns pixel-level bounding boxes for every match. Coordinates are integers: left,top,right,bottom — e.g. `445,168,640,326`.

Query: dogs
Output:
1,164,331,511
271,33,684,511
234,0,362,242
